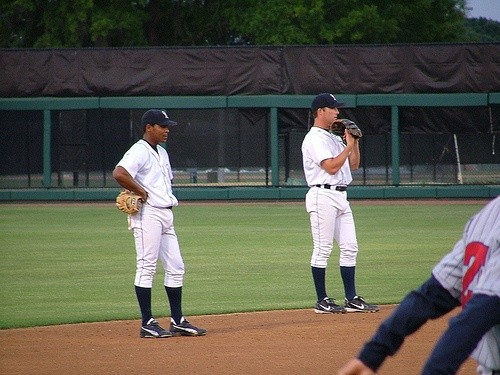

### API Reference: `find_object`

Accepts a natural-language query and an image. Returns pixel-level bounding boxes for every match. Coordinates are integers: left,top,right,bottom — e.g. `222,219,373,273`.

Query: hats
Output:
311,93,344,110
142,109,177,125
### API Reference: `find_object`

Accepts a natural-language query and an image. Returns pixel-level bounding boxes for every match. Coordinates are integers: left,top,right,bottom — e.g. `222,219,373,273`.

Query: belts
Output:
165,206,172,209
312,184,347,191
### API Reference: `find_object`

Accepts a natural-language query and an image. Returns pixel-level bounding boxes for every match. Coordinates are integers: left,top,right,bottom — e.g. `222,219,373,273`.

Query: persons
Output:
300,94,381,314
112,109,208,339
338,194,500,375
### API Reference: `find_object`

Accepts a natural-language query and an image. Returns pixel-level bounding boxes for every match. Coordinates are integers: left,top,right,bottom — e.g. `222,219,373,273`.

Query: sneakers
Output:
344,296,380,313
140,319,173,338
170,317,208,336
314,296,346,314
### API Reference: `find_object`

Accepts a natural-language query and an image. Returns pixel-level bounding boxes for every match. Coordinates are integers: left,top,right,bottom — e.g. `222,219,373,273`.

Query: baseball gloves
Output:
330,119,362,139
116,190,142,216
